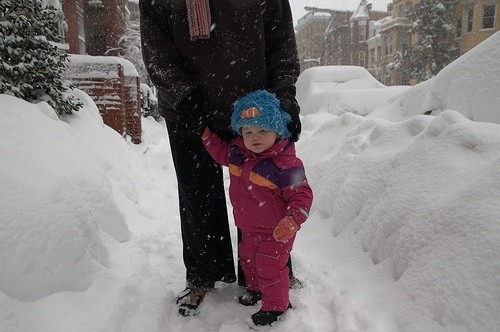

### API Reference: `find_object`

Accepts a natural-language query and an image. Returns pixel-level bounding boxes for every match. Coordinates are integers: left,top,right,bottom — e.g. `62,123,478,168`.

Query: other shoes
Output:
239,288,262,306
251,301,291,328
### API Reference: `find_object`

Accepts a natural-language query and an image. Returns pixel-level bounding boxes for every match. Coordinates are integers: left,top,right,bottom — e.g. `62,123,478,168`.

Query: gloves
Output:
272,216,298,243
280,96,300,142
177,92,230,137
199,127,211,142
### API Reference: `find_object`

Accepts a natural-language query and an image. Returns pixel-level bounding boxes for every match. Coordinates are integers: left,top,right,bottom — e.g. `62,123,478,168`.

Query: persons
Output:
138,0,305,317
194,89,313,328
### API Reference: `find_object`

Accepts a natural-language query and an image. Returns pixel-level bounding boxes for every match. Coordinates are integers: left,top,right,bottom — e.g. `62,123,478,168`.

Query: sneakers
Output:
288,277,300,289
176,283,204,316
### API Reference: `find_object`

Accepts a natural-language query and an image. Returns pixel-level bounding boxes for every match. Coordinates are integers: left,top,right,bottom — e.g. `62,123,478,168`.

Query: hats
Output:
231,90,292,139
